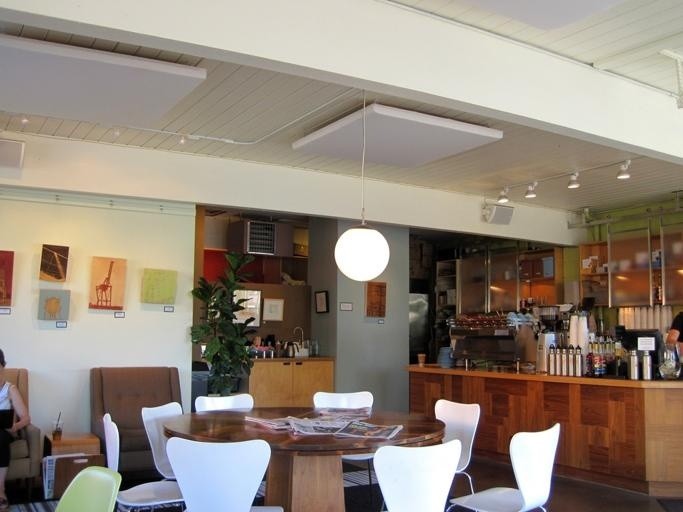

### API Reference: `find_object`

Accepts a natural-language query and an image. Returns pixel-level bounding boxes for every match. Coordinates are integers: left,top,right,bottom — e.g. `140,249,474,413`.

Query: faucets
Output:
293,326,305,349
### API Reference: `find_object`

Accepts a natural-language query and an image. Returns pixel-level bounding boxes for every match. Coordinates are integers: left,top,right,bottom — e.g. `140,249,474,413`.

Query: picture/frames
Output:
314,288,329,314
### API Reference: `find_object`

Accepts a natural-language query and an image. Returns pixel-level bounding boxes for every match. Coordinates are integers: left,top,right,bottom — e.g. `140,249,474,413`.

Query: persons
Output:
0,350,31,512
666,311,683,370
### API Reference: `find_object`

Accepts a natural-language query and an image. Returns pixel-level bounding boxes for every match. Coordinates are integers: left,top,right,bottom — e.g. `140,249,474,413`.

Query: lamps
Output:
332,89,392,282
288,101,505,169
1,31,209,128
497,157,632,205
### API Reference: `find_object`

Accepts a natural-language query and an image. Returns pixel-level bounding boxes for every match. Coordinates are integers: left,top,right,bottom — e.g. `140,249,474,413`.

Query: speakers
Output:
0,138,25,169
486,205,515,225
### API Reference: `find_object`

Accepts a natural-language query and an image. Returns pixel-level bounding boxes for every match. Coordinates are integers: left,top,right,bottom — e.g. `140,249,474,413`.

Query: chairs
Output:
54,466,123,511
444,422,562,511
372,439,463,512
195,391,255,414
0,366,44,502
137,402,187,480
165,435,284,511
431,399,482,512
89,366,185,479
312,389,375,509
100,410,186,511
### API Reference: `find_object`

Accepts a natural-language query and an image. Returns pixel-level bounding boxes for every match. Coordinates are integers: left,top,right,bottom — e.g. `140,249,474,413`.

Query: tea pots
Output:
438,346,456,369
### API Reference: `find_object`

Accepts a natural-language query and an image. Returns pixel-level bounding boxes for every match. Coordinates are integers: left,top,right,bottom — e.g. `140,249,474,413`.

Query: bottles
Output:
653,273,657,302
548,340,623,376
596,307,604,333
439,287,447,306
657,274,662,301
641,350,653,380
627,349,639,380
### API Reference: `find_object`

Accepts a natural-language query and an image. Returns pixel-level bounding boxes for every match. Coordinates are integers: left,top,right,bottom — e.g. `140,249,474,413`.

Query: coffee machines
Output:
535,306,564,373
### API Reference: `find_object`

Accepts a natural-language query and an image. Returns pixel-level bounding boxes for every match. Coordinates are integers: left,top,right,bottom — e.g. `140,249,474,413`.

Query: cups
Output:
570,315,588,340
582,280,591,292
51,421,64,442
521,295,547,308
660,343,681,379
455,313,532,329
286,341,299,358
603,249,662,273
617,305,673,334
464,358,472,369
417,353,426,366
243,344,282,358
311,335,319,356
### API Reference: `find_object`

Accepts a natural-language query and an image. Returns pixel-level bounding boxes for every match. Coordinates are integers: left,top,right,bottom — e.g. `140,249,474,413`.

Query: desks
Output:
162,406,448,512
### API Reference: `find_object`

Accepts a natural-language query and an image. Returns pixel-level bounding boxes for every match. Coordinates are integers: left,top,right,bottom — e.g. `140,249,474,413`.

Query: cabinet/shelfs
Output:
43,431,101,458
576,224,683,311
237,355,335,407
200,246,309,291
433,246,563,325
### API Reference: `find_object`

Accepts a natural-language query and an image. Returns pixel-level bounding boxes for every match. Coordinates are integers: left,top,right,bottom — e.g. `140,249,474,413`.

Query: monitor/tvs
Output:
624,329,667,364
